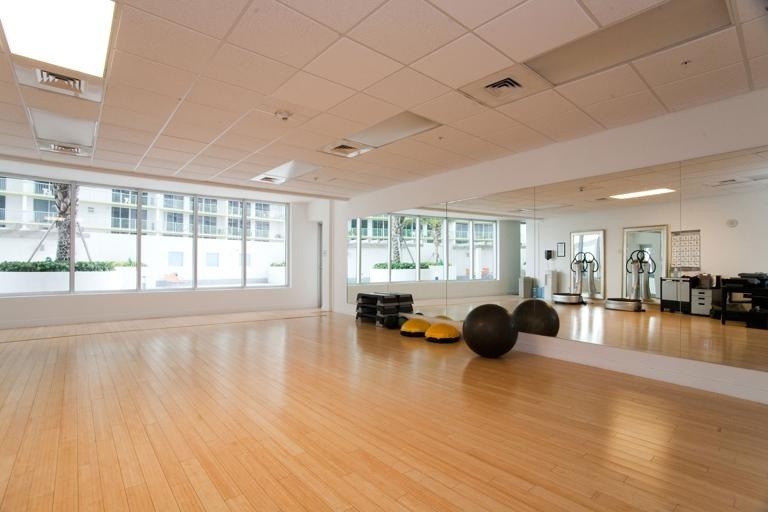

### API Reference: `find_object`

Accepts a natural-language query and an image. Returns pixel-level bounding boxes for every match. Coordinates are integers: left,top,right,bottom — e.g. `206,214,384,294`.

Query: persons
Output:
479,265,493,279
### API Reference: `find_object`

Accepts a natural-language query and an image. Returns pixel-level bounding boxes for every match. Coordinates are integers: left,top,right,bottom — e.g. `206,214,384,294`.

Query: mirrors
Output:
345,143,768,372
568,231,604,299
622,226,664,300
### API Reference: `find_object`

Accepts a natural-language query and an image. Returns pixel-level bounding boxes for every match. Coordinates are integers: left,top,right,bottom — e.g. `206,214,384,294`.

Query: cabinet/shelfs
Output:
658,275,690,313
688,287,722,316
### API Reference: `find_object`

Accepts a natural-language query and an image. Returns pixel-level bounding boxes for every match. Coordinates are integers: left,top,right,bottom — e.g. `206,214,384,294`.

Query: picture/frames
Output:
556,242,565,257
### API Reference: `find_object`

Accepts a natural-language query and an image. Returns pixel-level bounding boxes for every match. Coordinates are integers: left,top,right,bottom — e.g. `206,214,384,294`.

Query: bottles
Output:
673,268,678,278
